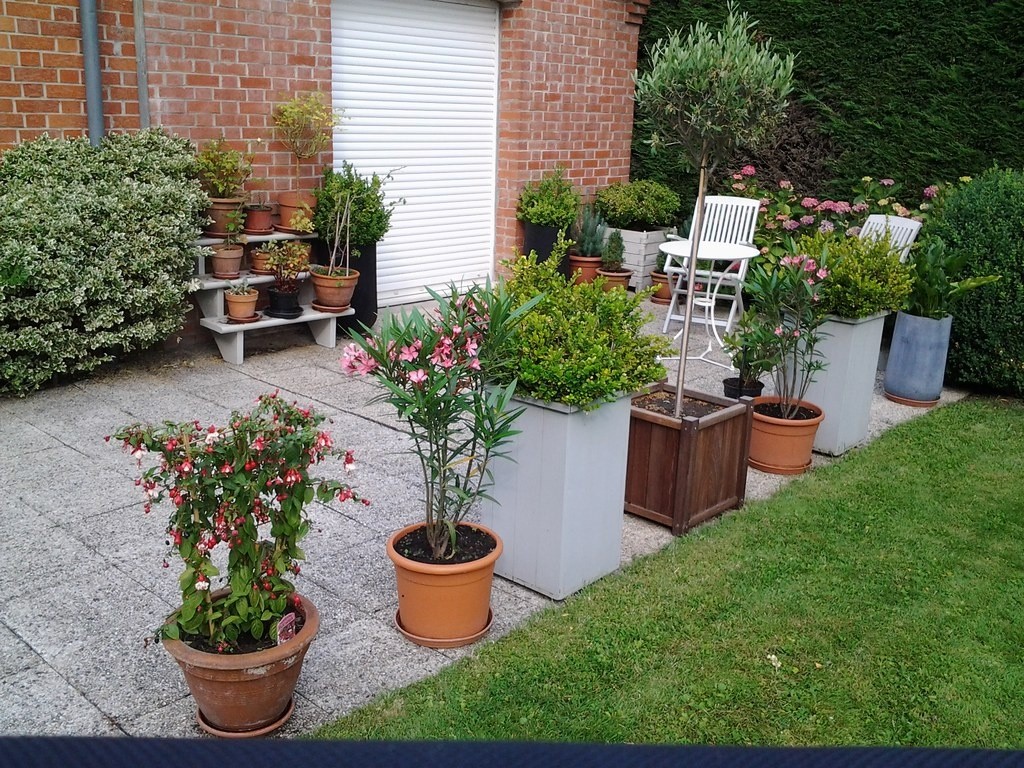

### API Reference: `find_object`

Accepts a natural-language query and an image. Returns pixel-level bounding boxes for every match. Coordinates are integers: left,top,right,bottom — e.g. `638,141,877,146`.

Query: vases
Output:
382,525,504,650
158,586,320,740
748,399,831,477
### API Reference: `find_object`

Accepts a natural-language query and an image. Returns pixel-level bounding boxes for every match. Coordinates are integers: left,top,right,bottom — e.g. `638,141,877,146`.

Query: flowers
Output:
102,390,369,640
743,252,829,416
342,294,538,525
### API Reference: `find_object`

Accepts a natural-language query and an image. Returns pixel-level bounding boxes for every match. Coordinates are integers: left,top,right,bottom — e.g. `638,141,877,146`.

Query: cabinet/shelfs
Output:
184,234,357,364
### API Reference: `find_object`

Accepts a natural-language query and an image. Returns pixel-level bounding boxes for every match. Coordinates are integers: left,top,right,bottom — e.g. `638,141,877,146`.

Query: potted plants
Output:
881,240,1006,407
780,235,912,458
516,165,581,280
651,244,683,308
593,231,630,301
568,202,605,290
185,88,359,322
723,305,774,402
592,178,683,302
620,0,800,539
476,239,666,603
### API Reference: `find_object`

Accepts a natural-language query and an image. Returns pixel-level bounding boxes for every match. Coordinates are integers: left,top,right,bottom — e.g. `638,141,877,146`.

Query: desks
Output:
654,239,763,370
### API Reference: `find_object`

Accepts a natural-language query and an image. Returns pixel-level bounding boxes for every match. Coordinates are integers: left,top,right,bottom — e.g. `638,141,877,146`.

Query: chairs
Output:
661,193,759,341
857,212,923,266
